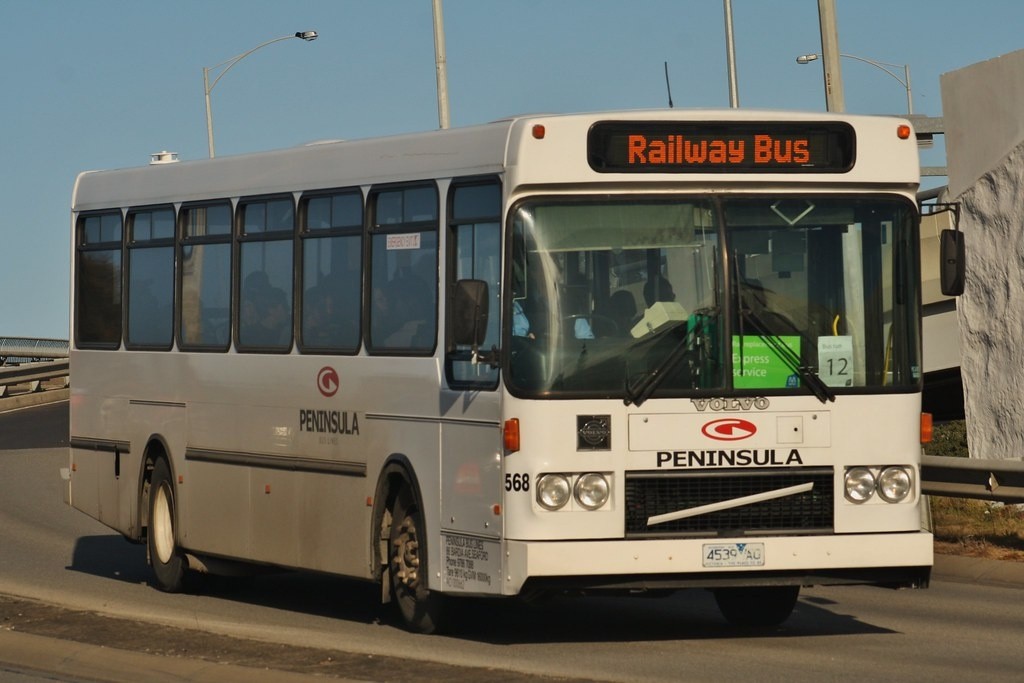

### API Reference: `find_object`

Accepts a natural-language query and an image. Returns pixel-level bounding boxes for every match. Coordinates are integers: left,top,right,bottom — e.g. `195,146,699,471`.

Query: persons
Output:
746,277,798,335
513,252,595,339
610,290,636,322
642,276,675,308
241,270,387,348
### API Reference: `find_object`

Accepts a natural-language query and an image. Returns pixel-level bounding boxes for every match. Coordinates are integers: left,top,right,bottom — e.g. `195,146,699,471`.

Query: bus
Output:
59,108,966,635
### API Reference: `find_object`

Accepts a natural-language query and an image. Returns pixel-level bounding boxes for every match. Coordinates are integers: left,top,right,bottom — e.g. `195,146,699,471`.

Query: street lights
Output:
795,53,913,112
202,30,318,157
